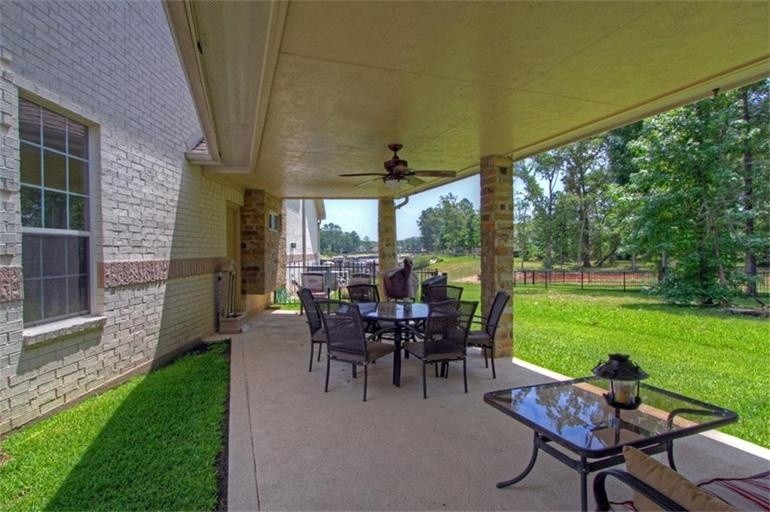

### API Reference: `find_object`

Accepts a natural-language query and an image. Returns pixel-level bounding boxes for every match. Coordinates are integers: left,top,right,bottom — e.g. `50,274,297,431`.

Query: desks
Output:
484,376,738,512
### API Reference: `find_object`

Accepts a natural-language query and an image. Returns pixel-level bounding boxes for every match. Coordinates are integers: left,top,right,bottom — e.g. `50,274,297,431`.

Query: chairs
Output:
296,269,512,402
593,446,770,512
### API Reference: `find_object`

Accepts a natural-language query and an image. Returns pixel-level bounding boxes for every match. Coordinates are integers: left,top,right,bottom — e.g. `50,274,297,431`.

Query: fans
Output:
338,143,457,188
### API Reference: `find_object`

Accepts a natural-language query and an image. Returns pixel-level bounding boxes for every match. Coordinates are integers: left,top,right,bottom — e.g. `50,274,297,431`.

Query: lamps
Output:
383,176,409,190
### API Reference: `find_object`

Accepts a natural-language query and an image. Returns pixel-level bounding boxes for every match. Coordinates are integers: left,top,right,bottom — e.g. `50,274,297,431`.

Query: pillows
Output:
622,445,745,512
